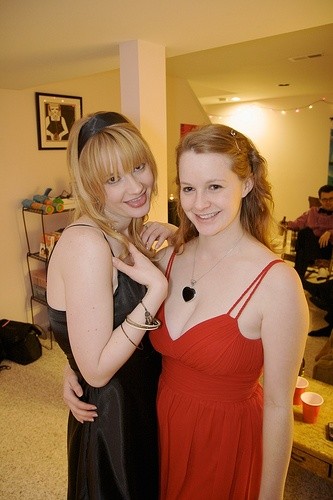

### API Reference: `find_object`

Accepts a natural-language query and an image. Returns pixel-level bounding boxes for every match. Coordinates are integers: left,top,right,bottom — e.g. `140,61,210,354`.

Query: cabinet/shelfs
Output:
22,206,75,351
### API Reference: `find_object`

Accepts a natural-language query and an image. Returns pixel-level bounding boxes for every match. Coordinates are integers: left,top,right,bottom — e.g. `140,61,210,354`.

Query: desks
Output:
290,374,333,484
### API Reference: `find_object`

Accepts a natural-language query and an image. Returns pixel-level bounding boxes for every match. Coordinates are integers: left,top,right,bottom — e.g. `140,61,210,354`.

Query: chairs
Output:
280,197,333,277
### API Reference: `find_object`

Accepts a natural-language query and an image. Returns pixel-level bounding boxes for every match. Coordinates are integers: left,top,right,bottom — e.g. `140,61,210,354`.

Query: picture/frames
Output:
35,92,83,150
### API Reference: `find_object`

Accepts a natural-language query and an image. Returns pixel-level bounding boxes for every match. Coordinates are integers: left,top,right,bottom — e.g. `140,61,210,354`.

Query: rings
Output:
144,224,148,229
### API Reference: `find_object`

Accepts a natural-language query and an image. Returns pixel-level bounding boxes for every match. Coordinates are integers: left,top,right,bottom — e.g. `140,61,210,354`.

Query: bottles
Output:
278,216,286,235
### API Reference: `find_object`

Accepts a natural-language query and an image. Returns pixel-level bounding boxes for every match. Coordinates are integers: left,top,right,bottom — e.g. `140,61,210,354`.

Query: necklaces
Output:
182,233,247,303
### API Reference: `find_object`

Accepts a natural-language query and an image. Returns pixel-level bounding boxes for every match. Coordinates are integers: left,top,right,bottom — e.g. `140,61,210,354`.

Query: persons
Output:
43,112,178,500
60,123,310,500
281,184,333,385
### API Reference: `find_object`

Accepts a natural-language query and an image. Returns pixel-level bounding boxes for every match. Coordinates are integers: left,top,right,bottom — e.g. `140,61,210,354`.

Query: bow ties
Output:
318,208,333,215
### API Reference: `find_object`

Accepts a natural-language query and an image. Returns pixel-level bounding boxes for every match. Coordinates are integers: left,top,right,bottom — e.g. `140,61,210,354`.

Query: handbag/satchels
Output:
0,319,43,365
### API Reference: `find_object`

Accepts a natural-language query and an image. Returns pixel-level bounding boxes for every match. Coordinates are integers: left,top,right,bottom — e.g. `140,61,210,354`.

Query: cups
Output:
292,375,308,406
301,392,324,424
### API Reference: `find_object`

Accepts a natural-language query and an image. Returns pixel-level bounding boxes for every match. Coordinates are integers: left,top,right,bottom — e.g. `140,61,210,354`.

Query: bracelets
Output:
121,300,162,351
